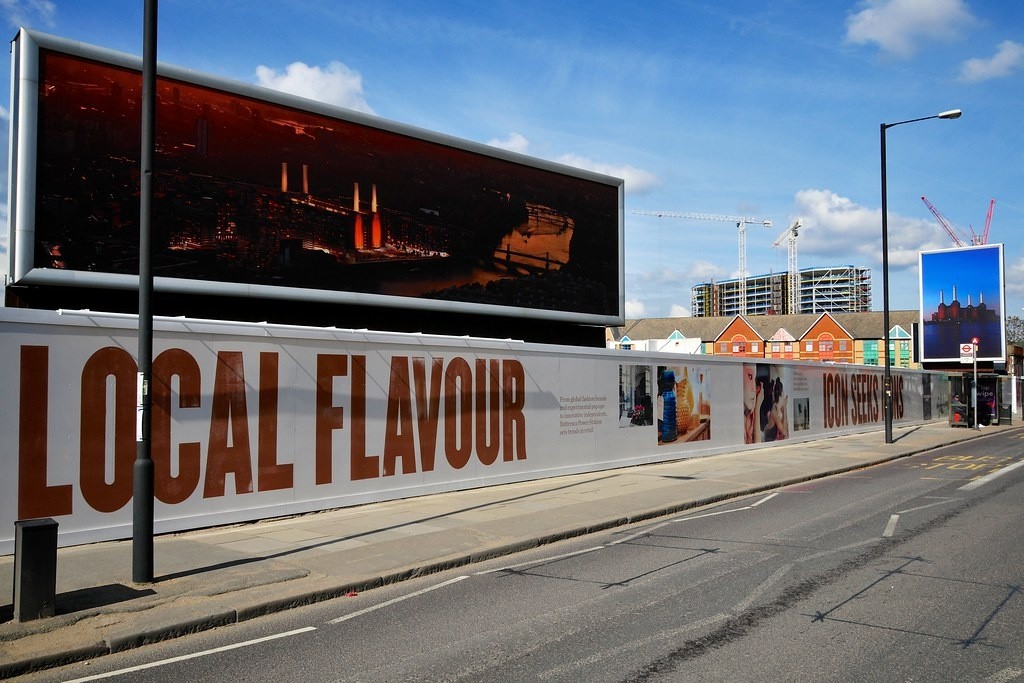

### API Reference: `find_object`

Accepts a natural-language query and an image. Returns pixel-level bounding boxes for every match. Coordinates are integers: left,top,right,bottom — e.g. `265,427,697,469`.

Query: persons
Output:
742,363,790,446
952,394,967,422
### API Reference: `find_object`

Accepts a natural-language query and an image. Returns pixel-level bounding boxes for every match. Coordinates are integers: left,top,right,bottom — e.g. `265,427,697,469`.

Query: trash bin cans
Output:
998,404,1011,426
